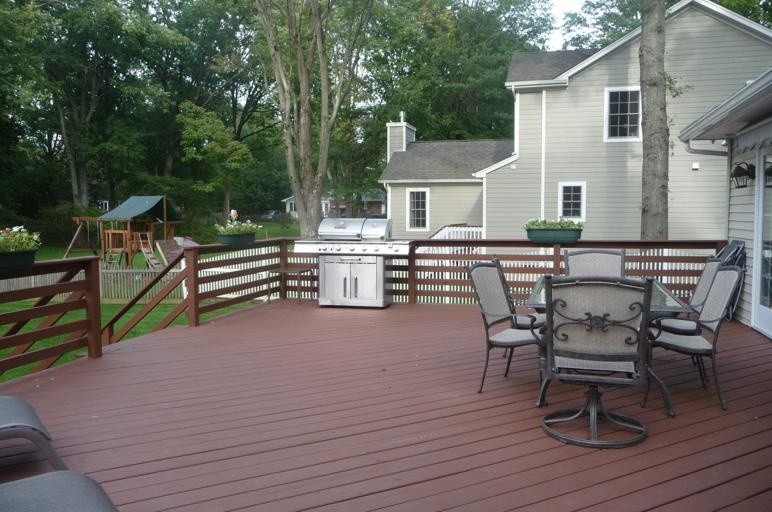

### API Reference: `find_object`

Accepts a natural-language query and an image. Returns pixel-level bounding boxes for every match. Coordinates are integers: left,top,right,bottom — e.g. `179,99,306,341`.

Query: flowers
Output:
215,209,263,234
522,216,584,230
0,225,42,252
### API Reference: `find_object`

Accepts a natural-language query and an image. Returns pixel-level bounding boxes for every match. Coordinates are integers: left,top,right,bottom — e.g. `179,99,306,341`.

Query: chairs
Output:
704,240,745,323
539,273,659,449
488,253,550,381
561,248,628,280
640,260,725,409
464,260,549,395
639,263,742,417
0,395,119,512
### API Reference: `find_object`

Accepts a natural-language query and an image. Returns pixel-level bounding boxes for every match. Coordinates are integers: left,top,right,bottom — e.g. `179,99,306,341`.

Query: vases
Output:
525,229,583,244
0,250,36,274
216,233,255,244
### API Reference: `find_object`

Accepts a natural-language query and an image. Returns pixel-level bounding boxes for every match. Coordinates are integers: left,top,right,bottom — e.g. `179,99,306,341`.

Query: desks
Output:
268,267,313,304
524,273,696,420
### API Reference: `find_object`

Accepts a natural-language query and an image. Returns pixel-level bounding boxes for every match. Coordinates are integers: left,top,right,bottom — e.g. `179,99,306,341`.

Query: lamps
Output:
730,161,755,189
